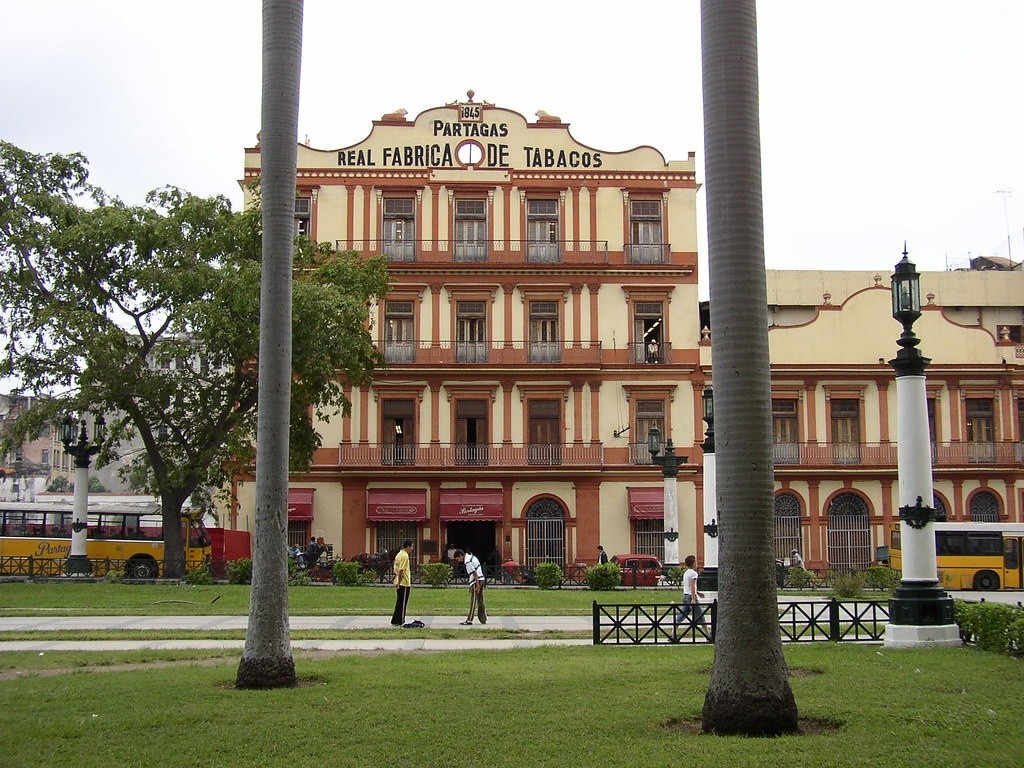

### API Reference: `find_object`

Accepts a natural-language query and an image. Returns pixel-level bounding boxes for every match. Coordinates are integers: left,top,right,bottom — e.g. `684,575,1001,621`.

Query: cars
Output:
610,554,662,586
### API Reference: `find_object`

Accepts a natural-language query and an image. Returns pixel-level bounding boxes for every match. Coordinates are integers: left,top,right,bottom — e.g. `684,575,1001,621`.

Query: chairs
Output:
0,524,147,540
937,544,984,555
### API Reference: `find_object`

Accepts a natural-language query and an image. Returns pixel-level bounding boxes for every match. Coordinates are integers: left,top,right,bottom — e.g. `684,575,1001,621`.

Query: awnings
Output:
627,487,664,521
287,488,314,522
439,489,503,521
367,488,427,522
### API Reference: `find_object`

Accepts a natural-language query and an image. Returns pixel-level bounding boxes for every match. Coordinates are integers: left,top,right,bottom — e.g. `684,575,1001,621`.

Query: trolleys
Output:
776,559,795,588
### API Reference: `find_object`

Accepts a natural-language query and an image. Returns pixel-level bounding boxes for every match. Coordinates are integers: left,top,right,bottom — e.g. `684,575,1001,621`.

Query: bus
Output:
0,500,211,579
888,521,1024,592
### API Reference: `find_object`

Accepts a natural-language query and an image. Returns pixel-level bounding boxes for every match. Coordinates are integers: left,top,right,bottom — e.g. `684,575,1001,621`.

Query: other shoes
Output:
460,621,472,625
668,637,680,643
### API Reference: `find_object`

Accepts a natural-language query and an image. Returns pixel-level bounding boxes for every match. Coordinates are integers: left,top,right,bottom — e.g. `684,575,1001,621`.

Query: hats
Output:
451,544,454,546
791,549,797,553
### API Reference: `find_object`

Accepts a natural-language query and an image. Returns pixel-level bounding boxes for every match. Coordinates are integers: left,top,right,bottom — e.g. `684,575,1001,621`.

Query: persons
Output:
448,543,457,560
648,339,658,359
391,539,415,626
596,545,609,565
791,549,805,569
667,556,711,642
293,536,319,562
502,556,518,584
453,549,487,625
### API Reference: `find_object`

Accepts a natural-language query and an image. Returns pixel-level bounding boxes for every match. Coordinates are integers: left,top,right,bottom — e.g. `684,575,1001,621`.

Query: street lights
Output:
884,252,965,647
697,381,719,591
648,424,679,575
59,412,107,583
